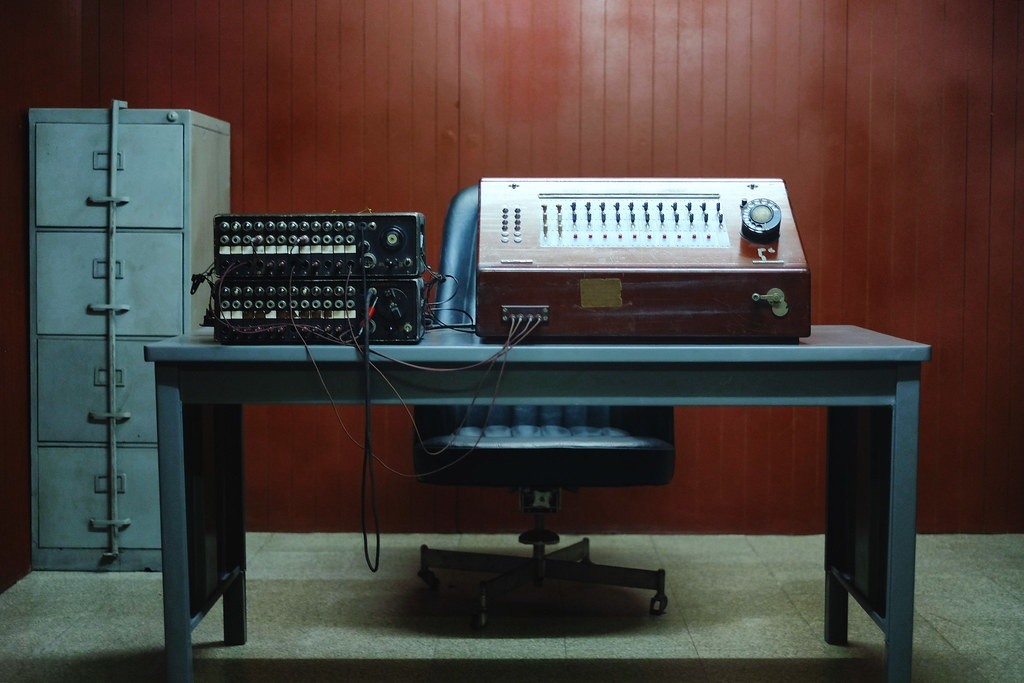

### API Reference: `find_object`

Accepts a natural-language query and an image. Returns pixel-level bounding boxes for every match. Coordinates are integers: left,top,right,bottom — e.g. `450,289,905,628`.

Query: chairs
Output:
413,183,675,632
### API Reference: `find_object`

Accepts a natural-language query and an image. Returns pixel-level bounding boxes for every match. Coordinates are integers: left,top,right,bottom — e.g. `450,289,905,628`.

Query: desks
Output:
144,324,932,683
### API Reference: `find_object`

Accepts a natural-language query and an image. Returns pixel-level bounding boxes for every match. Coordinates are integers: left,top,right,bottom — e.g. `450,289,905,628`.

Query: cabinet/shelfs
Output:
28,101,232,574
